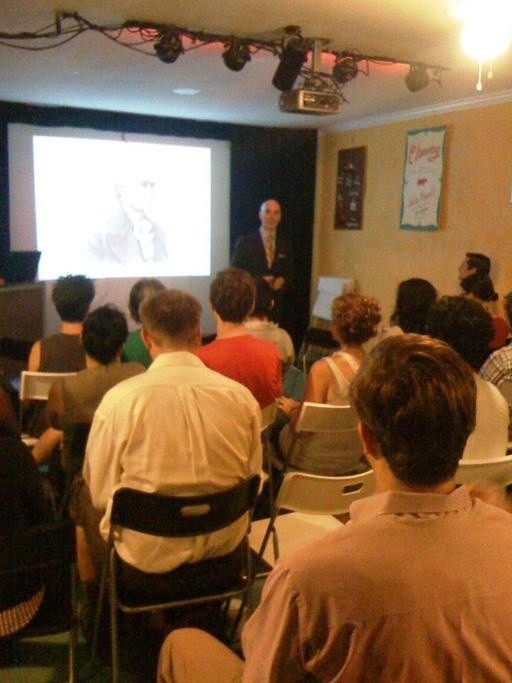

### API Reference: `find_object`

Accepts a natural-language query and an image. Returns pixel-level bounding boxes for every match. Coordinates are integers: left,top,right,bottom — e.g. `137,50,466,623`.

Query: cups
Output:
220,598,247,649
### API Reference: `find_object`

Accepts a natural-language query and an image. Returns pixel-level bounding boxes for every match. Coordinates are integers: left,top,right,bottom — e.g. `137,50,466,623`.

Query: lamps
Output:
332,51,358,84
272,26,311,92
223,40,250,72
154,32,184,63
405,62,430,92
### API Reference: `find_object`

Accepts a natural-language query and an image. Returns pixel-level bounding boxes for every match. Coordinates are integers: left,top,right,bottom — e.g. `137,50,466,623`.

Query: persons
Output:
31,269,295,482
0,382,52,656
272,294,382,479
71,289,263,638
384,252,512,465
230,196,294,328
154,332,512,681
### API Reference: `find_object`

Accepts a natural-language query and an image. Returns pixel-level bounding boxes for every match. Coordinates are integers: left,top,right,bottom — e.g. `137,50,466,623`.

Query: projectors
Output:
280,89,342,116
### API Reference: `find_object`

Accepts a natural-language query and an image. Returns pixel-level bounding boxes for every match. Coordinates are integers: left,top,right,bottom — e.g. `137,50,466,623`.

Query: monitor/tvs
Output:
0,250,42,287
